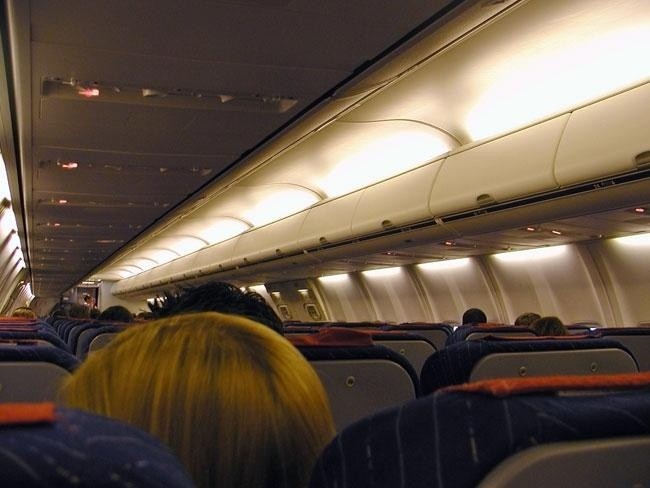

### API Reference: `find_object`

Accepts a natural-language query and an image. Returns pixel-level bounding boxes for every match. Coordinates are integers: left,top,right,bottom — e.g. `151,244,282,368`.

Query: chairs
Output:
0,313,650,487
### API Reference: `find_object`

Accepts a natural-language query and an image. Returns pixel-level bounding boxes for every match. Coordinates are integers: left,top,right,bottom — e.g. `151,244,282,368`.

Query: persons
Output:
528,315,570,336
13,279,287,340
55,312,336,487
514,313,542,327
462,307,487,326
